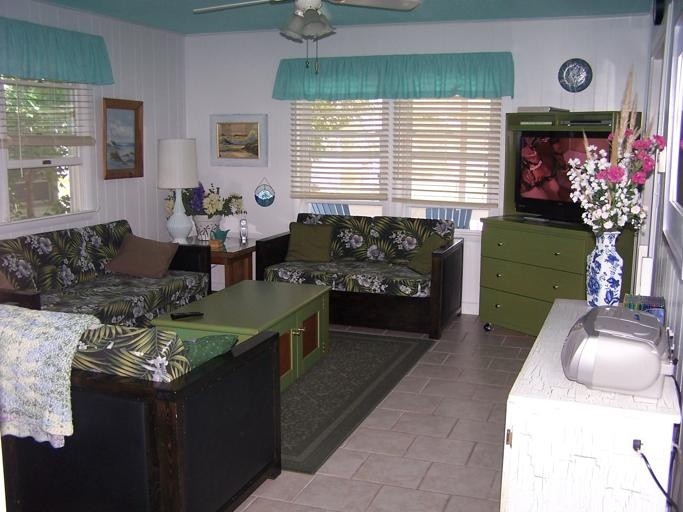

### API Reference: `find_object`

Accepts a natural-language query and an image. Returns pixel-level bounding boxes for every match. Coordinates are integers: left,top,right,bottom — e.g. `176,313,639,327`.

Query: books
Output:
621,292,666,314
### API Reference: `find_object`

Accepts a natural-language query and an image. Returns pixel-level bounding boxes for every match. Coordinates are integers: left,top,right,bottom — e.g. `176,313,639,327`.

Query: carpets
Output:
279,330,436,474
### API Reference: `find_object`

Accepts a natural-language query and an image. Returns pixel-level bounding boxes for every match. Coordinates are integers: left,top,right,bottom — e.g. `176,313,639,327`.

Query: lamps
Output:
157,137,200,245
279,0,336,43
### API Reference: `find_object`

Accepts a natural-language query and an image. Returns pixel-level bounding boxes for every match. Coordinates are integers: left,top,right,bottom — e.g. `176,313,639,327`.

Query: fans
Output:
193,0,419,23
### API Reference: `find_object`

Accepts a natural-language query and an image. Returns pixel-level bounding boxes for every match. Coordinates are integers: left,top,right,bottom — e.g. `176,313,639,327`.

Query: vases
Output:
192,215,222,241
585,229,623,308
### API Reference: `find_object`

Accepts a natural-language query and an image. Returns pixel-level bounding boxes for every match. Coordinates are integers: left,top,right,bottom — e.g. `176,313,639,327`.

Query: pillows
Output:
181,334,239,370
71,326,191,385
406,232,446,275
109,234,179,279
284,221,336,262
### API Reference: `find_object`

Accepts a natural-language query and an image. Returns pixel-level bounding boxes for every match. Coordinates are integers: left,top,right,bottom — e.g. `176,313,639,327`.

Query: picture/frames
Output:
209,113,268,168
102,98,143,180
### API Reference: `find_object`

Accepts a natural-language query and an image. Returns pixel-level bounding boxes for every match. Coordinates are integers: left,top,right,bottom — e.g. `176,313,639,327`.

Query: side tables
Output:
169,234,256,292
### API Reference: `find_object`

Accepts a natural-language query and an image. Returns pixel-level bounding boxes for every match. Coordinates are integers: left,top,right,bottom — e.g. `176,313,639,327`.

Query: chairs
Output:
2,331,281,512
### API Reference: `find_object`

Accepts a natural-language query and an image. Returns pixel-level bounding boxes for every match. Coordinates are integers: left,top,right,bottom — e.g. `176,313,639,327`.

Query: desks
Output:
479,214,634,338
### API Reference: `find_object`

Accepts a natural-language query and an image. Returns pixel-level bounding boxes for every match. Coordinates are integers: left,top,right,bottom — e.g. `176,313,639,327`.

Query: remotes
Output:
171,312,203,319
522,216,549,223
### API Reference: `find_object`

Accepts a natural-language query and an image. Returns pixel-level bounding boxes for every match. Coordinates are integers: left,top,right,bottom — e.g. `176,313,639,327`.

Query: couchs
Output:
256,212,464,340
0,220,211,328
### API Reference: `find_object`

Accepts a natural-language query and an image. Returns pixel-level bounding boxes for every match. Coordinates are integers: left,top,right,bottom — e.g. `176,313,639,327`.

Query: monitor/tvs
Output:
514,132,610,223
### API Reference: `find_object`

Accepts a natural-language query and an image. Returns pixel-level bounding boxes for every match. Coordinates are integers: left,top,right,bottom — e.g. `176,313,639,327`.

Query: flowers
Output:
567,128,666,237
163,181,247,219
